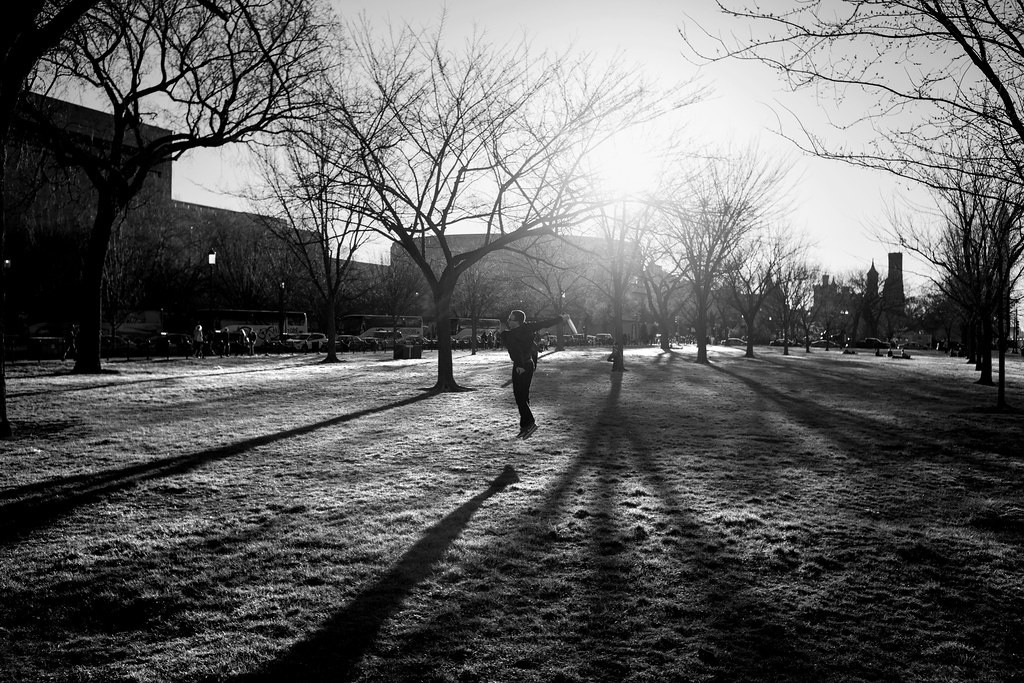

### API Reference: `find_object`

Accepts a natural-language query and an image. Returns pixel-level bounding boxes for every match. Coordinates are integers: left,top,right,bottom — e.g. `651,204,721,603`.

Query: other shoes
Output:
516,430,527,438
522,423,537,439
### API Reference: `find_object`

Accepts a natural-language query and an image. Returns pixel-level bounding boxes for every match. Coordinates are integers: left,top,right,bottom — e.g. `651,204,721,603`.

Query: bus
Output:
450,317,501,336
197,309,307,345
339,314,424,338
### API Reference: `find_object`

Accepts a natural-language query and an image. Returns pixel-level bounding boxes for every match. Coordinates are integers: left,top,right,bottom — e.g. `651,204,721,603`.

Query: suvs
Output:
286,332,328,352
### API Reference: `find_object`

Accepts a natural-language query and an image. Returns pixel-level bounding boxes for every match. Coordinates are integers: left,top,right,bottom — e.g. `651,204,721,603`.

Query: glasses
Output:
507,318,520,322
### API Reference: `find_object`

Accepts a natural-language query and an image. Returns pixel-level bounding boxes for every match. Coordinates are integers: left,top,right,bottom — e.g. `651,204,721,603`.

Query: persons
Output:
195,325,256,358
481,331,550,351
500,310,570,441
61,322,78,361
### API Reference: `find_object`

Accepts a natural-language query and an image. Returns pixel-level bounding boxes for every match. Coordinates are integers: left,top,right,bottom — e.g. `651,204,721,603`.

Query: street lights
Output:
208,247,217,307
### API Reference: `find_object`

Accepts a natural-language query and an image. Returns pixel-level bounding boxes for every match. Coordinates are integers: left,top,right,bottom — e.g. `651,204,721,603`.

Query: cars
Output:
770,338,892,349
728,338,745,345
63,322,255,355
936,340,967,357
897,341,928,351
266,333,387,352
406,332,611,348
